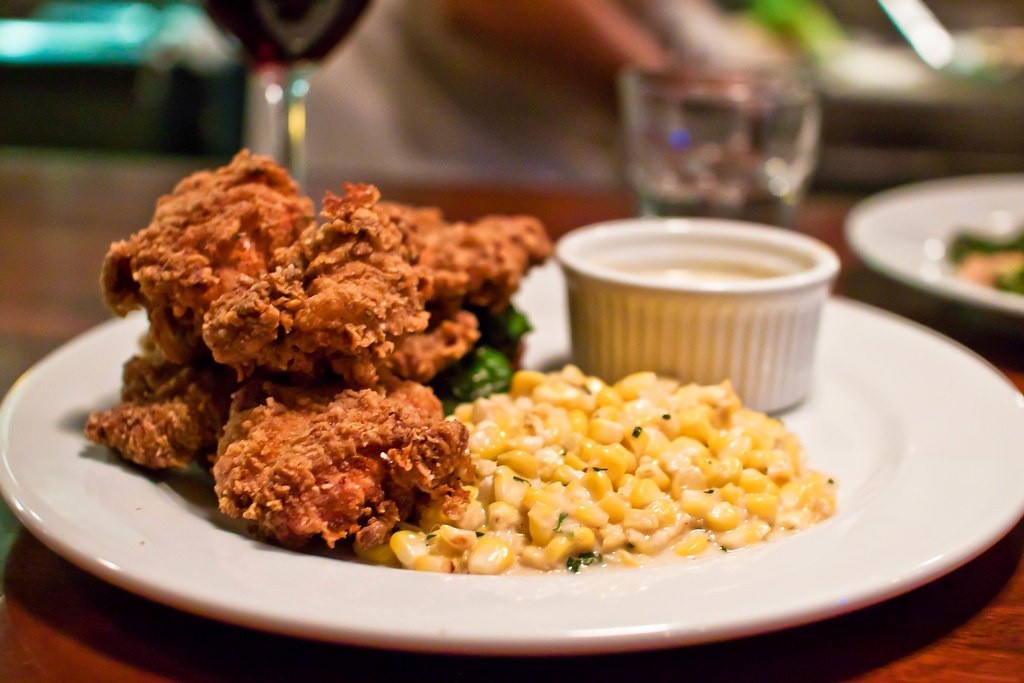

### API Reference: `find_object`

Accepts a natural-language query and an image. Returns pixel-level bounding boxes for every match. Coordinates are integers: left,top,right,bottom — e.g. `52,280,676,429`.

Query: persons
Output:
291,2,770,186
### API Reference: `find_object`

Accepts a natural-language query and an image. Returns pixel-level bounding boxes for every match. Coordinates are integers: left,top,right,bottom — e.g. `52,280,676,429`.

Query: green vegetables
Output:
432,301,533,416
946,222,1024,295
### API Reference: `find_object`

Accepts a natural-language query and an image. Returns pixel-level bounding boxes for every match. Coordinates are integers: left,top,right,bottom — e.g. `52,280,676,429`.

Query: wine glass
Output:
194,0,379,184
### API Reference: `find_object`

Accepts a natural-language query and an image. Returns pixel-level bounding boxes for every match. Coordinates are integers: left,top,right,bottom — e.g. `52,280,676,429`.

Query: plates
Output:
0,261,1024,654
845,171,1024,318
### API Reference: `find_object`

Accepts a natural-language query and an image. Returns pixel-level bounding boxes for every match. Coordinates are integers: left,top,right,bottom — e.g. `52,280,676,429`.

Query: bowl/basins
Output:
554,217,842,414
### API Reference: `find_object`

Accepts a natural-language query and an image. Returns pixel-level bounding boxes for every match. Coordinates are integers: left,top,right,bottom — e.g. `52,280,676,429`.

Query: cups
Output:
617,50,820,232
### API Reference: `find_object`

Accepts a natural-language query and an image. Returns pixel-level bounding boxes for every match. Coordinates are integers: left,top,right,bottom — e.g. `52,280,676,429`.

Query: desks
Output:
0,152,1024,683
